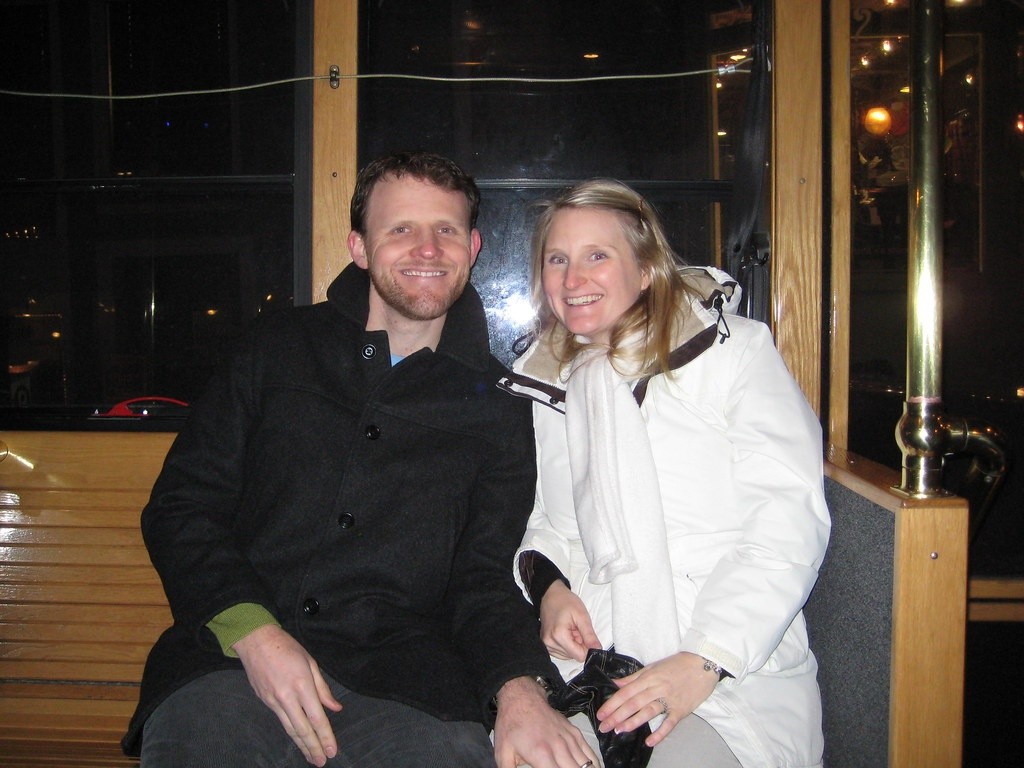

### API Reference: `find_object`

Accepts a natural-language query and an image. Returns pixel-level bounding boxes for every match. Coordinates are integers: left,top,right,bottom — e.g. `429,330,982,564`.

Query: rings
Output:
653,697,669,714
580,760,593,768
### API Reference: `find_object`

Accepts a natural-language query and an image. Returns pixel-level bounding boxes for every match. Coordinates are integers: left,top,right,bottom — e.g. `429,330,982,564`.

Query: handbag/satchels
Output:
549,648,653,768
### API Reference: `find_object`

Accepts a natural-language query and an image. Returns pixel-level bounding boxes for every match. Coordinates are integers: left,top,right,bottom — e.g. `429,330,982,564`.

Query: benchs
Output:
0,431,179,768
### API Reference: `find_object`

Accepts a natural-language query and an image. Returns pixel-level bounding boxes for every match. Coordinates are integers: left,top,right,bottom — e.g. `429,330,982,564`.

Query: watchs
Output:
527,674,553,691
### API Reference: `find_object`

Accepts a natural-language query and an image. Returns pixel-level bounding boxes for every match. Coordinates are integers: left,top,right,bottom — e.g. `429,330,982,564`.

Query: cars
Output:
87,396,190,419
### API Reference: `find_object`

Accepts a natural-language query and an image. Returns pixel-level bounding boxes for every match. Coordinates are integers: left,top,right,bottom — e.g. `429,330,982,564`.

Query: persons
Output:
120,149,603,768
488,174,833,768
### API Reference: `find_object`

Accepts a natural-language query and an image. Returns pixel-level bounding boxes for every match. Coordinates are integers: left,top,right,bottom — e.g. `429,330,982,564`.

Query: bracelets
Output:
702,656,722,674
